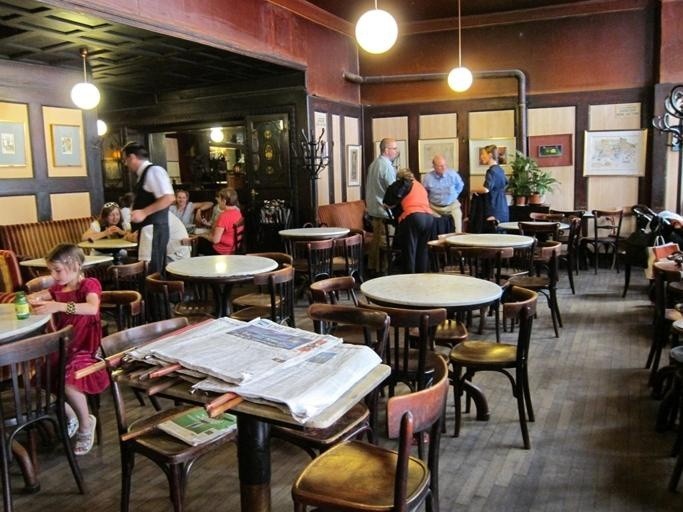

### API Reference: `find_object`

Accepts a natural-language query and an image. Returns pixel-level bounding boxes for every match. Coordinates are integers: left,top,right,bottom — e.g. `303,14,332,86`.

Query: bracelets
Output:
65,301,76,315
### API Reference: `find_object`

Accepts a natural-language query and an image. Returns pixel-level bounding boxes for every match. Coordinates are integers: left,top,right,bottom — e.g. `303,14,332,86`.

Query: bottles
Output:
14,291,31,320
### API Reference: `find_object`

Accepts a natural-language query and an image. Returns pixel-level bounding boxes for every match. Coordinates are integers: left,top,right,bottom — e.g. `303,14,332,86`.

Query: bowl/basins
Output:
186,224,196,234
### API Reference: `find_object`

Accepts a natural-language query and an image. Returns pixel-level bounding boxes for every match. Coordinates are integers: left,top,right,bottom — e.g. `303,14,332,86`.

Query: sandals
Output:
67,414,96,455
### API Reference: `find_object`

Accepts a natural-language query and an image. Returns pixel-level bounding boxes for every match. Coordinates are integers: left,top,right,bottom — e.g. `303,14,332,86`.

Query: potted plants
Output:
507,150,541,206
528,171,562,204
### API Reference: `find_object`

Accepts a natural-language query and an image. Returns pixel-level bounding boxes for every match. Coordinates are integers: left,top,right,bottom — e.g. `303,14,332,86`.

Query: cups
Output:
505,195,514,207
29,297,41,310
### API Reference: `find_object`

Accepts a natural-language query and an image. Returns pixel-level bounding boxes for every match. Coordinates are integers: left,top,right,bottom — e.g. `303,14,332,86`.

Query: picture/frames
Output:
51,124,83,167
346,136,517,187
581,130,645,178
0,121,28,167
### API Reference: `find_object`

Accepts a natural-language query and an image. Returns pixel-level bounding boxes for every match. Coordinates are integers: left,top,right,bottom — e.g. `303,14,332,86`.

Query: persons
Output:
421,155,465,232
365,137,400,275
28,243,112,456
469,145,509,233
81,141,246,280
382,168,435,274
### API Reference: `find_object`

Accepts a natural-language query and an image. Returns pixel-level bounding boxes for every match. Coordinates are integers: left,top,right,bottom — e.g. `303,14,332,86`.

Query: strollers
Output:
625,204,682,302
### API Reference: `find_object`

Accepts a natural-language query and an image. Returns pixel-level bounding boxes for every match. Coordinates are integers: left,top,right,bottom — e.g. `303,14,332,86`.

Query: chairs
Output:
574,209,625,276
0,198,583,510
621,198,683,511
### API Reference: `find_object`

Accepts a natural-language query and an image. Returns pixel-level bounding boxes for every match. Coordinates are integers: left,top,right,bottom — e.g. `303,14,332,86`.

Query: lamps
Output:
93,121,110,138
353,0,399,56
209,125,227,146
447,0,474,93
70,48,101,111
